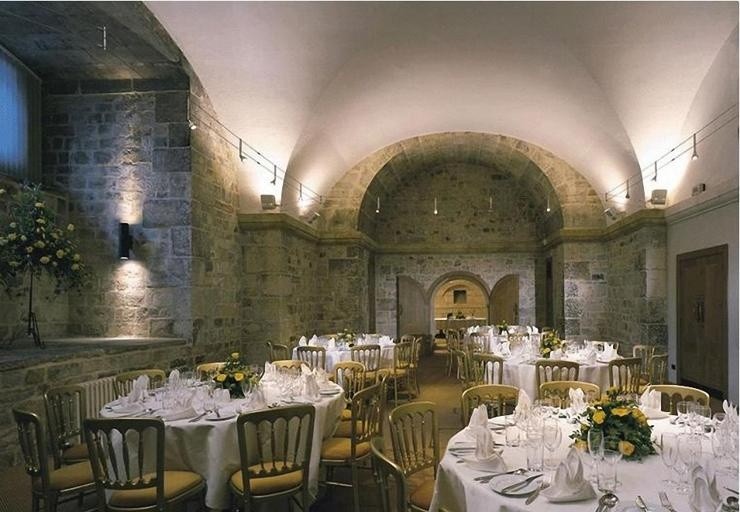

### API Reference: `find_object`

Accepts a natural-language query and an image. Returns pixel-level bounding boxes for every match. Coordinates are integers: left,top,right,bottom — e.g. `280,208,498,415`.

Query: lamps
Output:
261,195,280,212
188,116,195,131
118,222,132,261
646,189,667,205
603,206,626,227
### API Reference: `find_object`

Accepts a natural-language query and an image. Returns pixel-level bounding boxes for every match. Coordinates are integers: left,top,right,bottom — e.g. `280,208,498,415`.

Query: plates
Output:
109,379,341,421
490,473,538,495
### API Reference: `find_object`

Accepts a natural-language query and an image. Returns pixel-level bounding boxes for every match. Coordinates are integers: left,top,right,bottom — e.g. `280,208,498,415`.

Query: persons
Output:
455,311,467,320
444,312,453,339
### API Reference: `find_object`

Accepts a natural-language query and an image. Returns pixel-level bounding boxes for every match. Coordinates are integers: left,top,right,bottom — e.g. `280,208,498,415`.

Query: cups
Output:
504,415,520,447
595,450,617,492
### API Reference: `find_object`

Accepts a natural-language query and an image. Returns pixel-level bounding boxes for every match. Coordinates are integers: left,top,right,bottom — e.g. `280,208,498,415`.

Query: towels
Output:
168,369,180,389
474,427,494,459
553,446,583,490
688,465,720,510
246,385,264,413
128,374,150,403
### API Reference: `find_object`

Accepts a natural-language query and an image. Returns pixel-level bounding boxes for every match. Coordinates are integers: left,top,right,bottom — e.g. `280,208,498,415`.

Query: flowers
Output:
213,353,262,383
569,389,662,462
0,178,97,305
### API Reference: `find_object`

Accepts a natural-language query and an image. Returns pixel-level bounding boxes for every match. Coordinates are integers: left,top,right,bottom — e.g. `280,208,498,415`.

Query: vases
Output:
221,378,245,399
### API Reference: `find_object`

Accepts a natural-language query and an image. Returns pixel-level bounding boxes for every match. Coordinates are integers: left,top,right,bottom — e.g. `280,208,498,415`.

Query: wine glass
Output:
526,417,562,471
587,429,624,483
710,413,739,479
676,399,709,445
661,431,702,496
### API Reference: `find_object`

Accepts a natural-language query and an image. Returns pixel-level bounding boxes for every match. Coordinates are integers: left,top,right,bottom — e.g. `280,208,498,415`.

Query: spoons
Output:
595,494,619,512
725,495,739,511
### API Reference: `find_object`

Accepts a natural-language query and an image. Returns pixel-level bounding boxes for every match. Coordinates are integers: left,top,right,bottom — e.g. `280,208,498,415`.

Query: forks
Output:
658,491,678,512
526,474,552,505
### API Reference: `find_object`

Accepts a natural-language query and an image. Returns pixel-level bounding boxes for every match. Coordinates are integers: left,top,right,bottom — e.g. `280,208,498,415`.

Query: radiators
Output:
75,369,138,443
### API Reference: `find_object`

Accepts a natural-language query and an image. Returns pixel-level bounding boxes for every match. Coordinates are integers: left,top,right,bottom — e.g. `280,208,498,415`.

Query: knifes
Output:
501,473,544,493
449,446,501,451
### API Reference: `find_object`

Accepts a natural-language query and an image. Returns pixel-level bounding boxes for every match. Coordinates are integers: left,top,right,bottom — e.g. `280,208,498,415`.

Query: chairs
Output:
197,363,244,377
227,405,315,511
266,330,440,511
11,409,106,511
432,324,710,428
82,418,208,512
112,369,166,396
43,385,100,470
